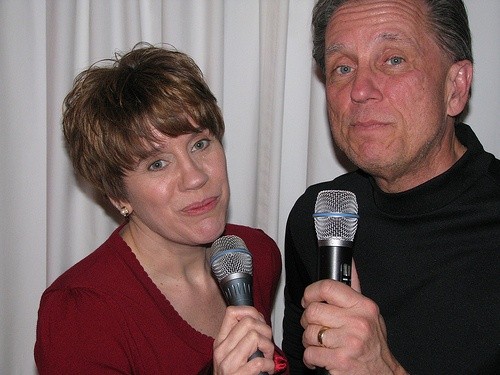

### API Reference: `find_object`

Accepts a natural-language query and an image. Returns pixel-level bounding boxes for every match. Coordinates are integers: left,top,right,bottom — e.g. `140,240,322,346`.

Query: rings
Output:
317,326,330,348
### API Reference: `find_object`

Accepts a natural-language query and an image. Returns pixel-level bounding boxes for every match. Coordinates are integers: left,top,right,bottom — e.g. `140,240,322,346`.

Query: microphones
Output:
210,235,270,375
313,190,360,375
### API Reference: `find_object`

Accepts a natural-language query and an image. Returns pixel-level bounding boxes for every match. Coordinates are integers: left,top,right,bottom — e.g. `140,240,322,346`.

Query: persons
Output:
285,0,500,375
33,44,282,375
